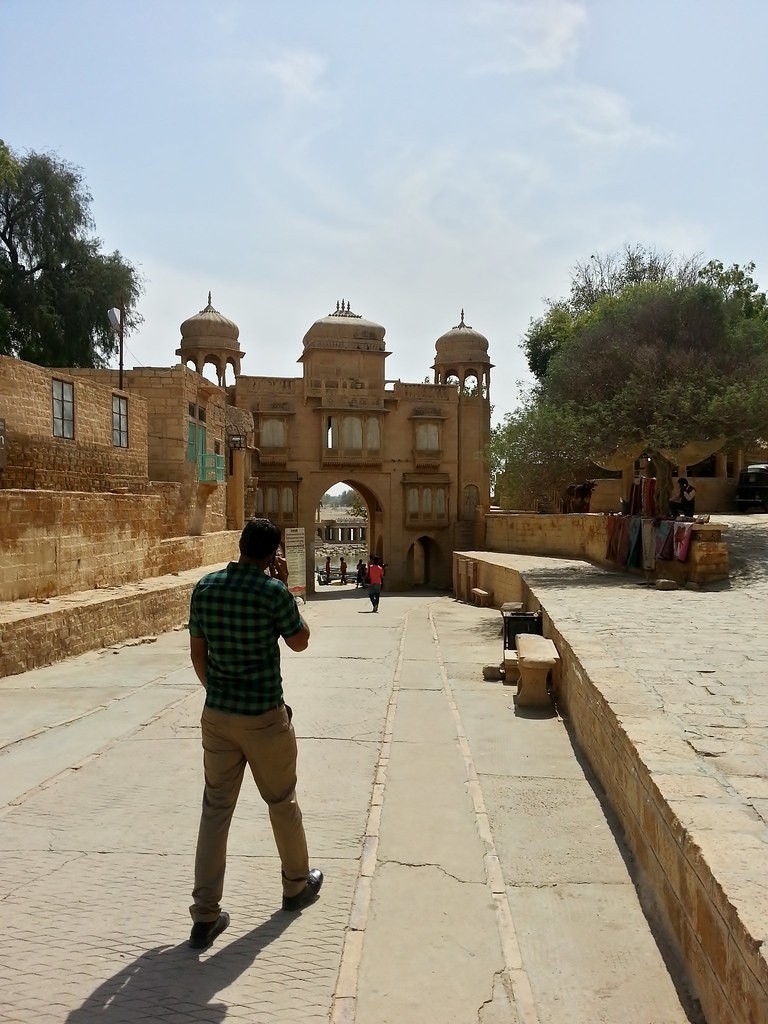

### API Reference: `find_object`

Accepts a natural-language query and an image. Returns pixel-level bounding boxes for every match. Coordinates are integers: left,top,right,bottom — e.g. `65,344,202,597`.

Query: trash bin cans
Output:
499,609,543,650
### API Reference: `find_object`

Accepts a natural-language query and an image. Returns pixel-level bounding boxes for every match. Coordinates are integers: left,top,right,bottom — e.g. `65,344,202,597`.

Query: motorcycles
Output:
734,463,768,514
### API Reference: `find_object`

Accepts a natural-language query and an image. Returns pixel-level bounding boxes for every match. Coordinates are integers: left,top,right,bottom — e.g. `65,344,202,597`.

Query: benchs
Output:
516,634,560,707
499,601,523,636
471,588,488,606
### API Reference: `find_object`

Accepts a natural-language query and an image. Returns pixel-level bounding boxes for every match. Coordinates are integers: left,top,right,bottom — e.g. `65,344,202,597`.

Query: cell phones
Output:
272,552,276,567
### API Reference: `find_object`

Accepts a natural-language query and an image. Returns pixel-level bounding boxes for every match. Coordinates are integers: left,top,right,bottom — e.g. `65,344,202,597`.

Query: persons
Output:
340,557,347,584
189,519,323,949
356,554,384,612
326,557,331,580
671,478,696,517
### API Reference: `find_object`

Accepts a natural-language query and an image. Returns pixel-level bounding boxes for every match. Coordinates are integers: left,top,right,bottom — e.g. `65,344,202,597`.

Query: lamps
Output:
227,434,247,449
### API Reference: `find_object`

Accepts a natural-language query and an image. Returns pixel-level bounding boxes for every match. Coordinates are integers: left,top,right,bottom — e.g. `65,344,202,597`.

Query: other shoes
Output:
373,604,378,612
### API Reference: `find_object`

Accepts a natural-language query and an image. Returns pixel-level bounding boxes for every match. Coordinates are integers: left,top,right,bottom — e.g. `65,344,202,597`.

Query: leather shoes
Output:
283,868,324,911
190,911,230,948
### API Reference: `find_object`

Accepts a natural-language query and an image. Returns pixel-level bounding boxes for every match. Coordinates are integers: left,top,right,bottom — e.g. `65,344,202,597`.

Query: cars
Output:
317,567,357,585
